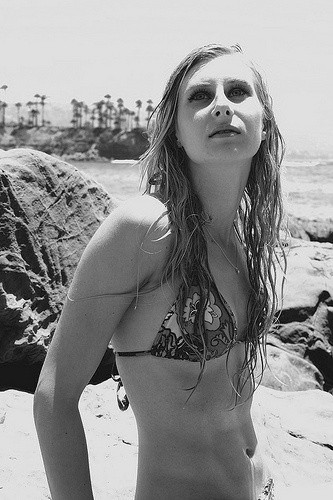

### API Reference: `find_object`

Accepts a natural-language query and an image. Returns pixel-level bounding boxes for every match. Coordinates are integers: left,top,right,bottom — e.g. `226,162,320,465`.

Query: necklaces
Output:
201,218,243,276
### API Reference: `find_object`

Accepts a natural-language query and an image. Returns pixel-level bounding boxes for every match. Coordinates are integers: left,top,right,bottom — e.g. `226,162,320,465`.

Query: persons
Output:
31,41,291,500
